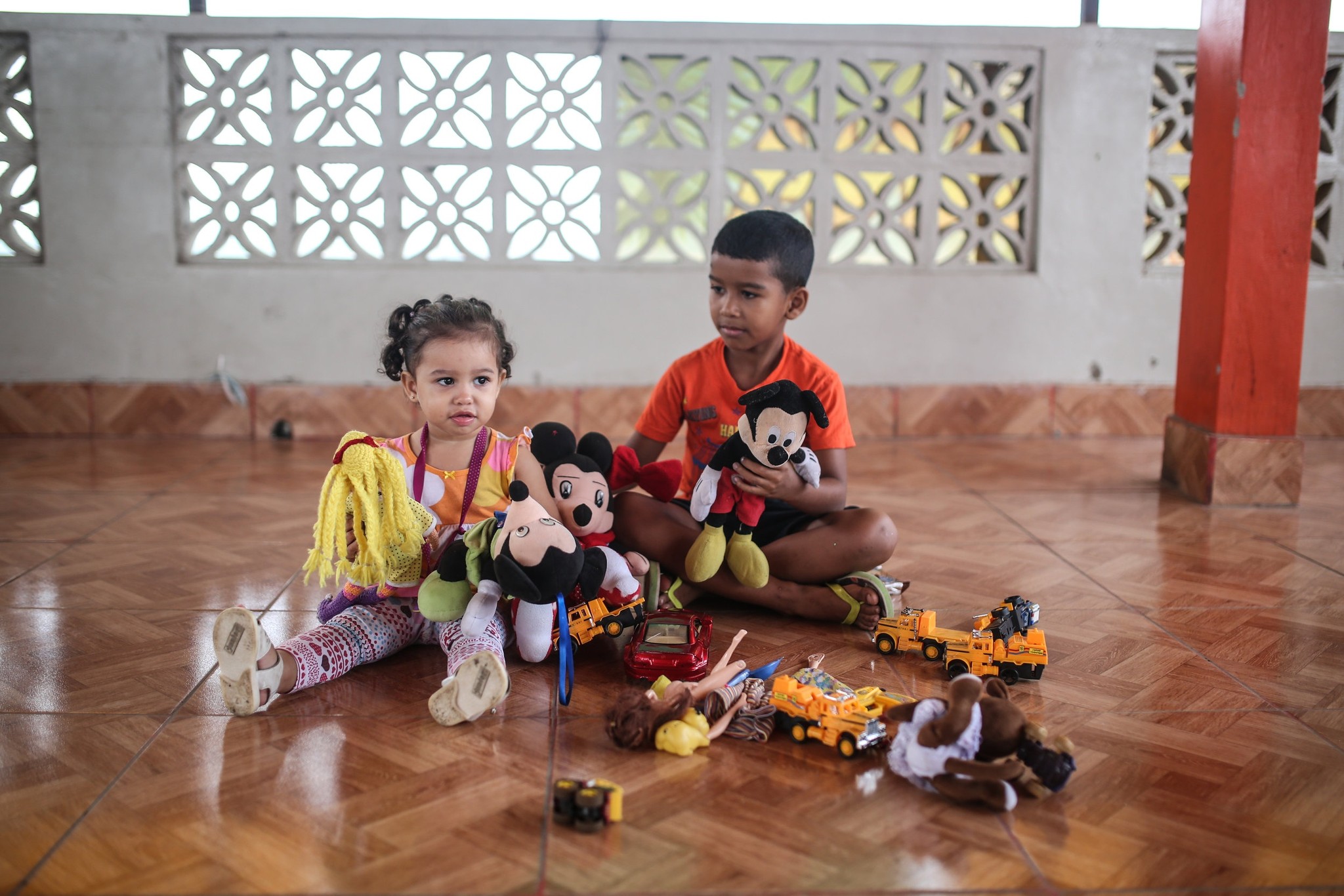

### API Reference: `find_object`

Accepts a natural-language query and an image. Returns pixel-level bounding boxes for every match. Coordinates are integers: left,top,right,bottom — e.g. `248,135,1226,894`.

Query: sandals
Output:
212,607,282,716
429,650,511,726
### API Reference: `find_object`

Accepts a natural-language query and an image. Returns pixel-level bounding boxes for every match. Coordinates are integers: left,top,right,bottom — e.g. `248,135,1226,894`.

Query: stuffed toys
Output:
886,674,1076,812
304,379,832,666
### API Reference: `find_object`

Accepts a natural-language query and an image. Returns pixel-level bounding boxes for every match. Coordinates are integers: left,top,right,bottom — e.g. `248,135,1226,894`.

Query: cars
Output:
623,607,714,684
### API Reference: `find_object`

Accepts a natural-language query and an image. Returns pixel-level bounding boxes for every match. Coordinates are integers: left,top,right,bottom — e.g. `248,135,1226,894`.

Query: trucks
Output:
972,595,1041,646
553,776,624,831
553,597,647,658
872,606,972,661
944,629,1049,684
769,668,918,760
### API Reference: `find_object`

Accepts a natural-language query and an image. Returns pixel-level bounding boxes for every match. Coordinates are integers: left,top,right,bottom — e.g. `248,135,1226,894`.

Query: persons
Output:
213,293,562,728
608,625,751,754
606,210,897,633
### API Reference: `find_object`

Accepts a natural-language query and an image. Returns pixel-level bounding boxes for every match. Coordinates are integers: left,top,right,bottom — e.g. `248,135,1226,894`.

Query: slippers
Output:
658,575,689,608
827,570,893,626
